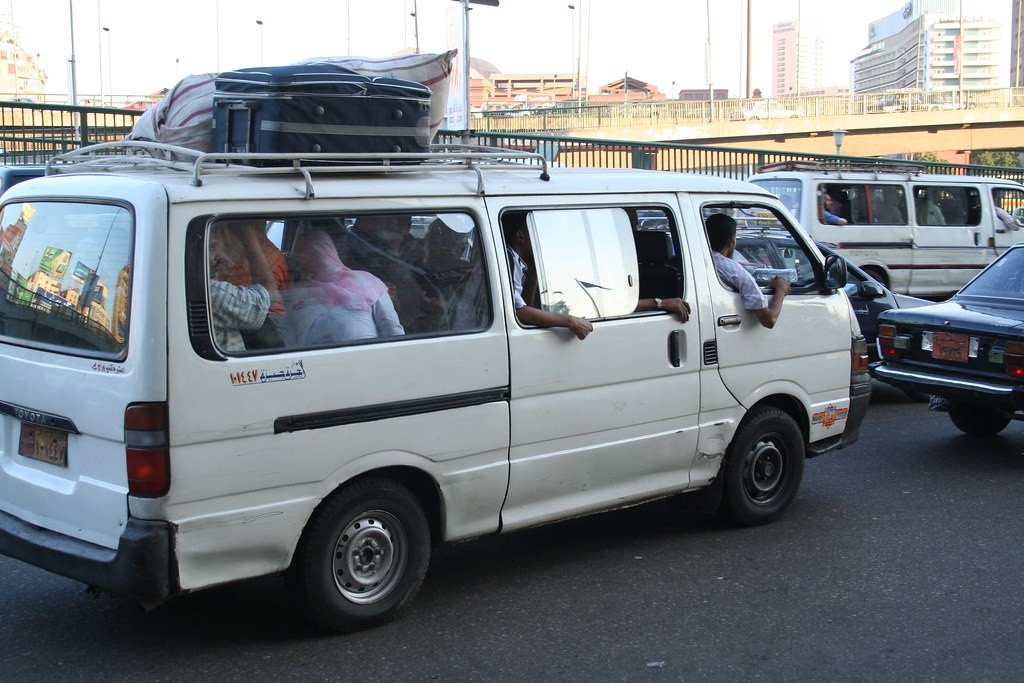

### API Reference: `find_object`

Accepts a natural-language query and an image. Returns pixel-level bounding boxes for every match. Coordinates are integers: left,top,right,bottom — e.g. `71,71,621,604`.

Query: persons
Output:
209,207,691,351
780,183,1020,230
705,213,791,330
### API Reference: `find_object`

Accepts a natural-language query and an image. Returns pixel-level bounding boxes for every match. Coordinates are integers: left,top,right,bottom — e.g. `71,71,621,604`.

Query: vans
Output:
728,160,1024,299
0,121,864,632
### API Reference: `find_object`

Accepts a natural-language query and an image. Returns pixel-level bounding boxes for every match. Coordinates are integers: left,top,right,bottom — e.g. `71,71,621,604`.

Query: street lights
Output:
563,3,580,112
452,0,500,145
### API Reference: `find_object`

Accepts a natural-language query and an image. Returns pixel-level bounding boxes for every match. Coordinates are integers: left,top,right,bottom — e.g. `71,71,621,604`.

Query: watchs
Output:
655,298,662,311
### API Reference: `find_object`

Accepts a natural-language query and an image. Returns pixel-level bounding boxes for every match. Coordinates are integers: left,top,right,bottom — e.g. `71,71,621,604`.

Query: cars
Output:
866,241,1024,435
0,164,71,205
716,223,938,403
